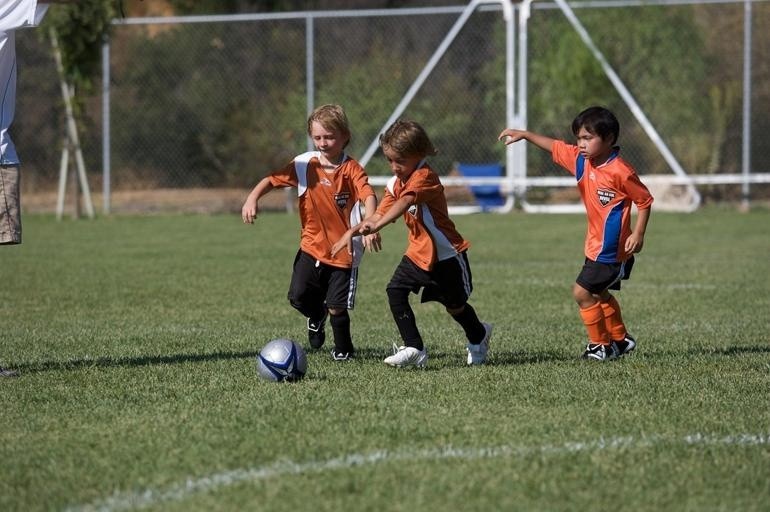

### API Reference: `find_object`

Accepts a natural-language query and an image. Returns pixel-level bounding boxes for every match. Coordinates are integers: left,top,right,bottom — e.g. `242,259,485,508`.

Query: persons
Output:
330,119,491,369
242,103,381,359
499,105,654,363
0,0,54,378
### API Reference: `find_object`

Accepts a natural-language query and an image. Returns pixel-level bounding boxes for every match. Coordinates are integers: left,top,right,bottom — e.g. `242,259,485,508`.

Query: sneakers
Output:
610,333,636,355
580,342,617,362
307,304,328,349
383,346,427,368
467,323,492,366
333,352,351,361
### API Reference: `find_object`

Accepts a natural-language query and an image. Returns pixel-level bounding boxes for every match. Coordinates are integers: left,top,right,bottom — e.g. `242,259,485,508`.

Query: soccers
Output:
256,338,308,382
